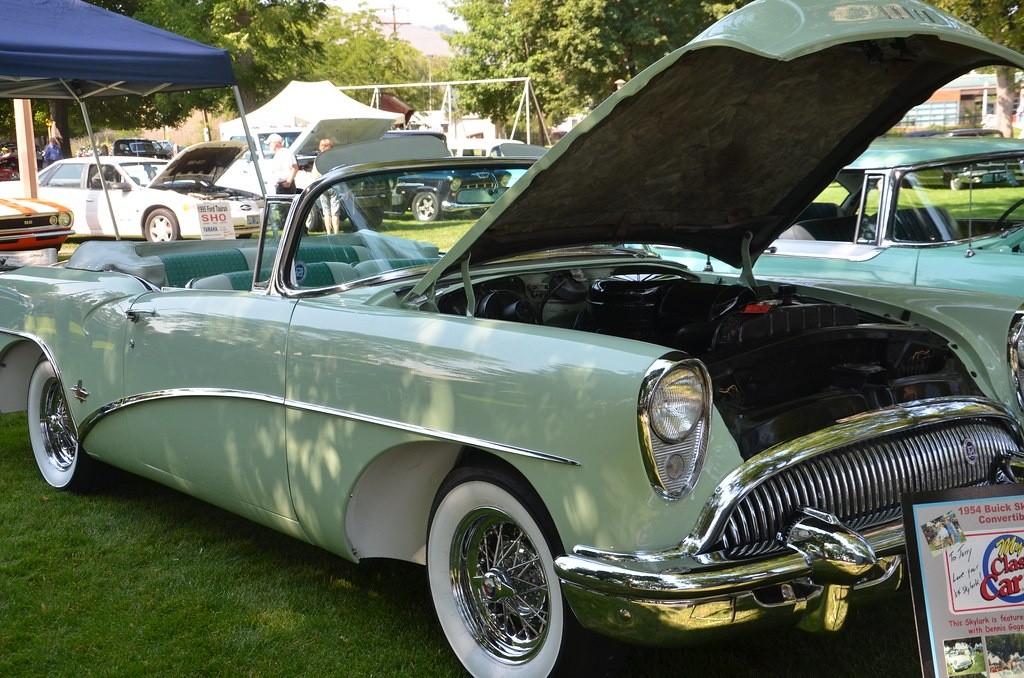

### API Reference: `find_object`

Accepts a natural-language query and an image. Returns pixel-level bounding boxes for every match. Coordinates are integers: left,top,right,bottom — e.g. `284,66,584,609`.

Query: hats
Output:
265,134,283,144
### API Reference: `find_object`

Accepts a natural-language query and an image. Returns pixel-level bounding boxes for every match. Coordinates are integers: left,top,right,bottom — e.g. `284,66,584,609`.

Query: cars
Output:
596,125,1024,437
0,0,1024,678
0,134,553,274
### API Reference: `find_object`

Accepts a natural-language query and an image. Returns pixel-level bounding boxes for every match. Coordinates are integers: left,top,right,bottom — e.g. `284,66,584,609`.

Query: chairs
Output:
862,205,961,241
777,203,871,239
154,244,442,289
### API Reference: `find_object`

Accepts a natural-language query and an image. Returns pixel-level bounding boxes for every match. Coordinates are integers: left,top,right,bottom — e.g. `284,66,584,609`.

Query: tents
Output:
218,80,405,142
0,0,280,243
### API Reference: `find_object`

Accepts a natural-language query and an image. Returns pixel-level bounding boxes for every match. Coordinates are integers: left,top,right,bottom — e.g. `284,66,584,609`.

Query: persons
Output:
263,134,309,236
45,138,64,167
936,517,961,546
312,139,341,235
